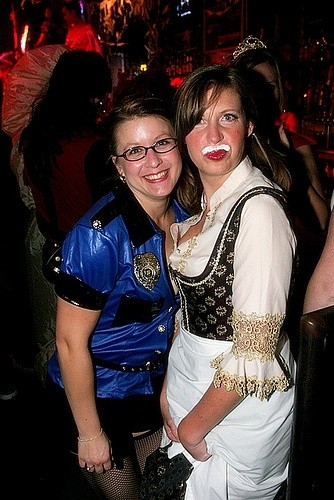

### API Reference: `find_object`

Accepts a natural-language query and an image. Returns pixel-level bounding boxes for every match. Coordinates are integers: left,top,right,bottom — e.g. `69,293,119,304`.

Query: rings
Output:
86,465,93,470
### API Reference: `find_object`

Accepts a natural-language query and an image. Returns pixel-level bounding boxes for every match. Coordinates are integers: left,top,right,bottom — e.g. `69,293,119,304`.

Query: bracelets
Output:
77,428,103,441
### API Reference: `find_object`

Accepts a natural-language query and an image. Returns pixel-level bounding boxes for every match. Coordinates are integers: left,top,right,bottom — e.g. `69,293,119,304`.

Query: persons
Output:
0,126,36,400
19,2,334,360
42,96,202,500
158,62,298,500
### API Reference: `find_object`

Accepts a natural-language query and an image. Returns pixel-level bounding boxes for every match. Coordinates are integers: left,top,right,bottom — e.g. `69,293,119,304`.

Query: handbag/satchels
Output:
141,448,195,500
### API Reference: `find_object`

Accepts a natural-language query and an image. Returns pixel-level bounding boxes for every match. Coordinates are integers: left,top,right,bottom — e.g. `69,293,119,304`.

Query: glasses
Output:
115,137,181,162
